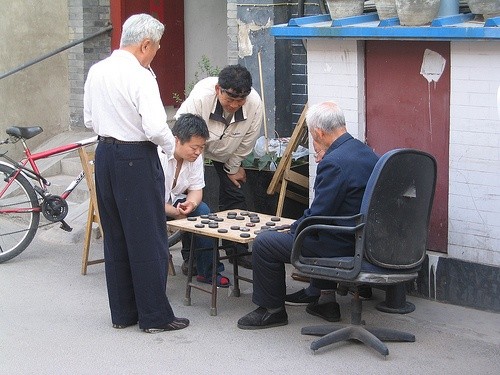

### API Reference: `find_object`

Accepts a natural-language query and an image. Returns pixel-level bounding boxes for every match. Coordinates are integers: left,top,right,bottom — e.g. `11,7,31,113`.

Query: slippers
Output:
198,274,230,287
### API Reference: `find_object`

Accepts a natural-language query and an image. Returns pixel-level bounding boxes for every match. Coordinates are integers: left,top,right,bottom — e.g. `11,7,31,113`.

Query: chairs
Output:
79,146,176,278
266,103,308,218
290,148,438,358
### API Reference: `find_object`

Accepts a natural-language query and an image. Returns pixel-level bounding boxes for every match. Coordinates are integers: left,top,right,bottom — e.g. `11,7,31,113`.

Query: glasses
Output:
221,88,251,98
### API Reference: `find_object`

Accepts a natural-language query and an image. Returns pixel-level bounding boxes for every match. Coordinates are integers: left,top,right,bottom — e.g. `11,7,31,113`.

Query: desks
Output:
168,209,298,317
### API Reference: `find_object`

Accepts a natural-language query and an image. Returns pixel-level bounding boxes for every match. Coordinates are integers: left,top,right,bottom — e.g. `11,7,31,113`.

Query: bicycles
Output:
0,126,98,263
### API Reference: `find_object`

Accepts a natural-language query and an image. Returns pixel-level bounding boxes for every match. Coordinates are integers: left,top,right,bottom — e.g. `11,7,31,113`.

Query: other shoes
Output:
145,318,189,333
285,288,319,305
229,253,253,269
306,301,341,322
348,287,373,300
182,258,196,275
114,320,137,328
237,305,288,329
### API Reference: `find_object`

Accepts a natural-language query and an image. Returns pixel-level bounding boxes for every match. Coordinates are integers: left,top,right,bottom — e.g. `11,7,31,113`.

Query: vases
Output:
324,0,500,28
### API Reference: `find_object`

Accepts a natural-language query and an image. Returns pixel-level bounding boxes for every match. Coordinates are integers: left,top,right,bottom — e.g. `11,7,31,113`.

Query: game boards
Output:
166,208,299,244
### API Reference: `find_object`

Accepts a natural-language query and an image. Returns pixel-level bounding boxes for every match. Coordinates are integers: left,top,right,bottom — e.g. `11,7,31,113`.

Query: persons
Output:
238,101,379,330
174,64,263,276
84,14,190,334
157,113,231,288
